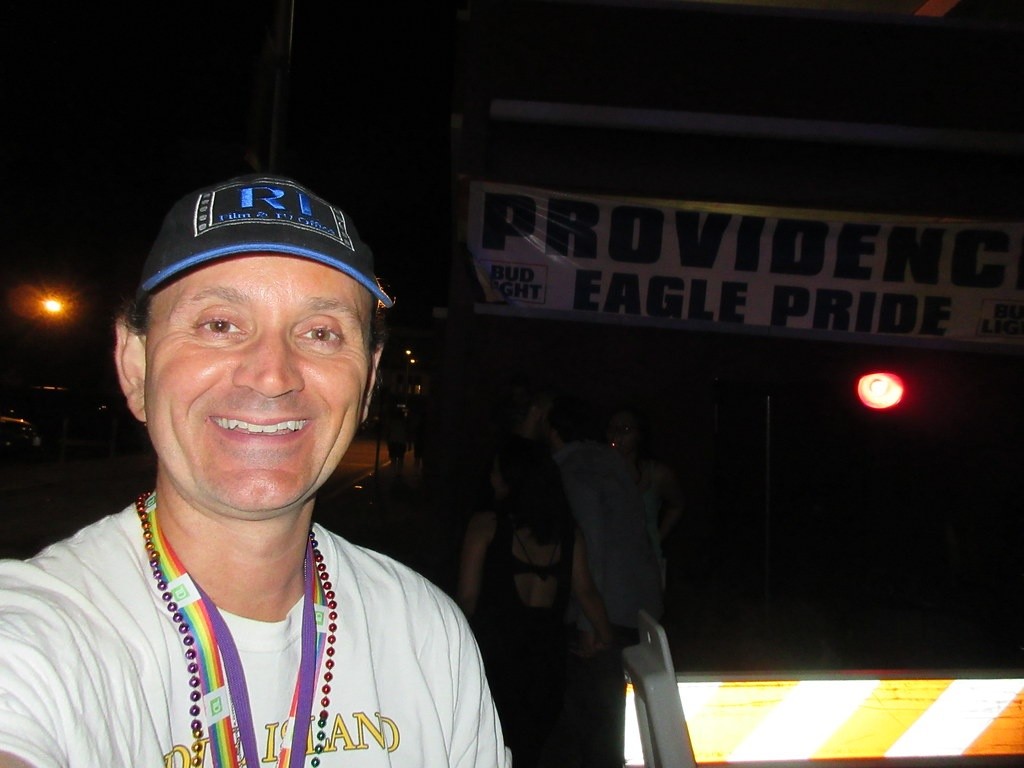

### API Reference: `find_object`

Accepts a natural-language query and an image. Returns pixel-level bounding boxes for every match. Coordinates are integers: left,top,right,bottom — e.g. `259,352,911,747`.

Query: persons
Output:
455,380,684,768
383,404,424,473
0,174,514,768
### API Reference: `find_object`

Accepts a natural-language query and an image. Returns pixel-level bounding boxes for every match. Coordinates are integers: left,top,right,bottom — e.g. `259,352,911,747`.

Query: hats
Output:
136,172,393,308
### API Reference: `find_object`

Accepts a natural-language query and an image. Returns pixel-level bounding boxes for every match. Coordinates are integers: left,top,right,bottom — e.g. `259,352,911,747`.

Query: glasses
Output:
606,424,639,435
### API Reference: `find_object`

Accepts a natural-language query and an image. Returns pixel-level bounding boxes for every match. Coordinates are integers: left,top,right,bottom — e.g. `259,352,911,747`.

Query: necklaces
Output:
132,490,337,768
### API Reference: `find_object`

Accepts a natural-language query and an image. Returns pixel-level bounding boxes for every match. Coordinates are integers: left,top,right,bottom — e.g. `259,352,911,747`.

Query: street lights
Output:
403,348,417,402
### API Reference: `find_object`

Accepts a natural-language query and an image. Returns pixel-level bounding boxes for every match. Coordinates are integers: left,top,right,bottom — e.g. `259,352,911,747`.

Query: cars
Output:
0,411,41,459
